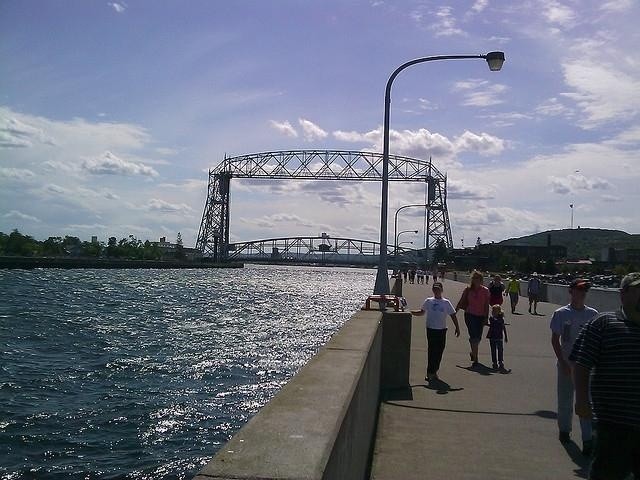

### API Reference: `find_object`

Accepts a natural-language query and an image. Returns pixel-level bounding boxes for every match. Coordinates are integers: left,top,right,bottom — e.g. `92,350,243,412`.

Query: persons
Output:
403,267,445,285
550,277,600,457
482,304,508,368
506,275,522,314
527,271,540,315
410,283,460,382
456,270,489,366
569,272,639,480
489,275,505,307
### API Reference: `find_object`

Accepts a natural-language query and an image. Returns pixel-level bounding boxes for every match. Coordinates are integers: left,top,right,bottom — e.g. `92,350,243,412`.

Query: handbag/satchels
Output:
458,296,467,310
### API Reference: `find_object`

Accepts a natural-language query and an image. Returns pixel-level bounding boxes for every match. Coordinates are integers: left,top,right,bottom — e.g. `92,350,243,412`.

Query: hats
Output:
432,282,442,290
569,278,592,290
530,272,539,277
620,272,640,291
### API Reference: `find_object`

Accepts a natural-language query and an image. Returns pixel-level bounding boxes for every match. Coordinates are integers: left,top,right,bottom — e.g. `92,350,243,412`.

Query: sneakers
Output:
582,440,593,457
424,374,439,384
559,431,570,445
470,352,478,361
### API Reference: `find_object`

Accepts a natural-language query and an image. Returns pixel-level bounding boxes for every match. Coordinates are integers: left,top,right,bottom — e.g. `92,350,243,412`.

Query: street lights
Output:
374,51,505,293
